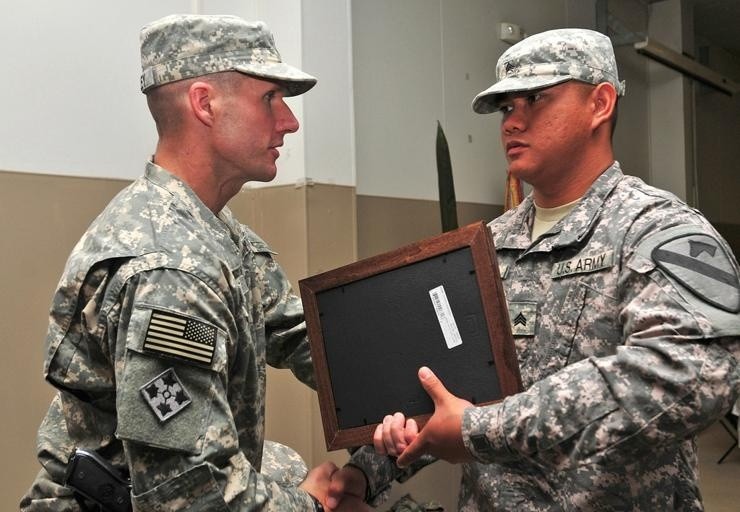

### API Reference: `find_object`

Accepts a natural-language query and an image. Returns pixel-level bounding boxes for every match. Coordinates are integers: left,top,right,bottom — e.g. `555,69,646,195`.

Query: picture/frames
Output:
298,220,522,453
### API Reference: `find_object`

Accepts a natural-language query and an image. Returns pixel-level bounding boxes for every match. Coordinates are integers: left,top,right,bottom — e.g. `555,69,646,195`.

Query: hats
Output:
137,12,319,99
471,26,628,115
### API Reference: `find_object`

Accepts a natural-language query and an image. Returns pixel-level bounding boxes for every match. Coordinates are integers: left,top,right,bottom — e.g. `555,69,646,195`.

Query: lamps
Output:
632,1,740,98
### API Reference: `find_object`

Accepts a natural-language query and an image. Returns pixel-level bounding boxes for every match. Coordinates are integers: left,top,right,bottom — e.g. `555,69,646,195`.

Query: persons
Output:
320,27,740,512
19,15,419,512
